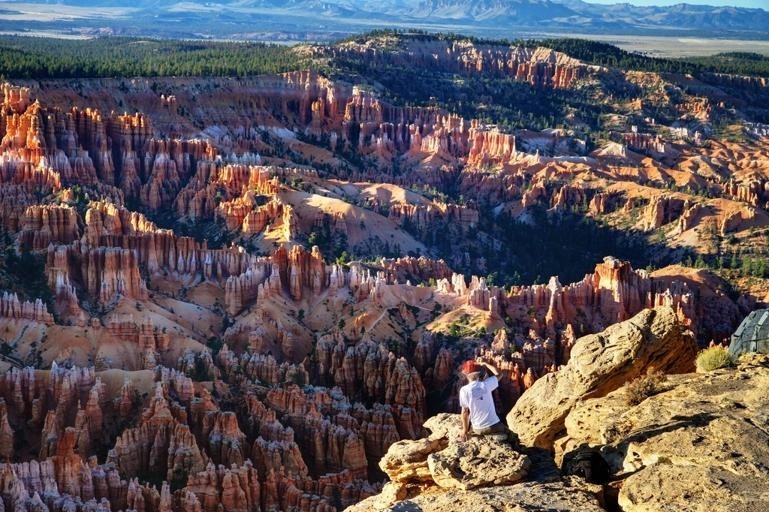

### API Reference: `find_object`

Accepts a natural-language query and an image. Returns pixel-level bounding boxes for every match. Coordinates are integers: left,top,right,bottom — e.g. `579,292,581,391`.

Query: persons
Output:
458,357,521,455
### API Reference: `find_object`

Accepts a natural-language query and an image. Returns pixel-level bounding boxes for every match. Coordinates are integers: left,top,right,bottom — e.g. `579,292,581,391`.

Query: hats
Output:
463,360,481,373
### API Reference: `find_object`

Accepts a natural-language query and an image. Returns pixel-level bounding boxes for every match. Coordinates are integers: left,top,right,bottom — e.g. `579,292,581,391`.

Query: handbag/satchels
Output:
575,448,610,483
